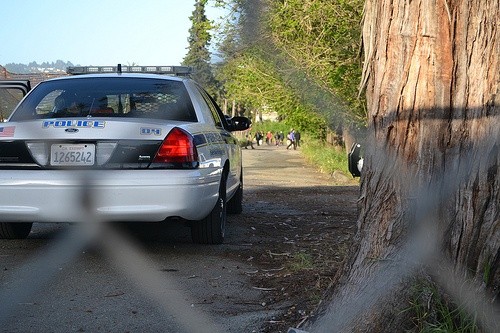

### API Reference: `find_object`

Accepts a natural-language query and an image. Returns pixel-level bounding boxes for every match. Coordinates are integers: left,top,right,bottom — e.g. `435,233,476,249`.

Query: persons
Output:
54,92,81,114
245,124,254,149
91,95,114,113
253,130,284,147
287,129,300,150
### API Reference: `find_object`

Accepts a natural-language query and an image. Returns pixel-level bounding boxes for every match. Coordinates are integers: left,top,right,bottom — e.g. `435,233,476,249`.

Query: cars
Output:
0,63,252,244
